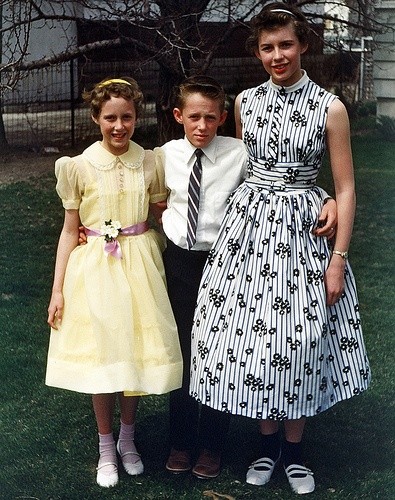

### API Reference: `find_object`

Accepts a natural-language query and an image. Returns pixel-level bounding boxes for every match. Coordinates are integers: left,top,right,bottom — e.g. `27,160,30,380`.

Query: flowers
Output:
100,220,122,244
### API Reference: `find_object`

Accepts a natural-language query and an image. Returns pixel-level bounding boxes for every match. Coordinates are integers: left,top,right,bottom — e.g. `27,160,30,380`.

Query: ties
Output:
187,149,204,250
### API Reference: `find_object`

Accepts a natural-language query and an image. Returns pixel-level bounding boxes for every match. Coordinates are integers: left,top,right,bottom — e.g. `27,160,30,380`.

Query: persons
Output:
155,4,371,495
45,77,184,487
77,74,338,478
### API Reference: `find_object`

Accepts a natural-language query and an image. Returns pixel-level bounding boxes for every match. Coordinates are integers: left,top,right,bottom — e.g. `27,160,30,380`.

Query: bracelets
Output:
323,197,333,204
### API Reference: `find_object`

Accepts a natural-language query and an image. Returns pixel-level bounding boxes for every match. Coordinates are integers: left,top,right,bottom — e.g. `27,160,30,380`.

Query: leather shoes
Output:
166,443,223,478
247,448,282,486
283,463,315,494
96,441,144,487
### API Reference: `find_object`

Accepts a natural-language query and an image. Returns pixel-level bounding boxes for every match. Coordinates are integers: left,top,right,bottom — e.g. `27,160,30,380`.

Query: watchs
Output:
333,251,349,259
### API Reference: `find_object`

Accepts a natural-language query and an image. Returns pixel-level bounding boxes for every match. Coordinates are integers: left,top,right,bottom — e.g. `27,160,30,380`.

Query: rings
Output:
330,227,334,231
